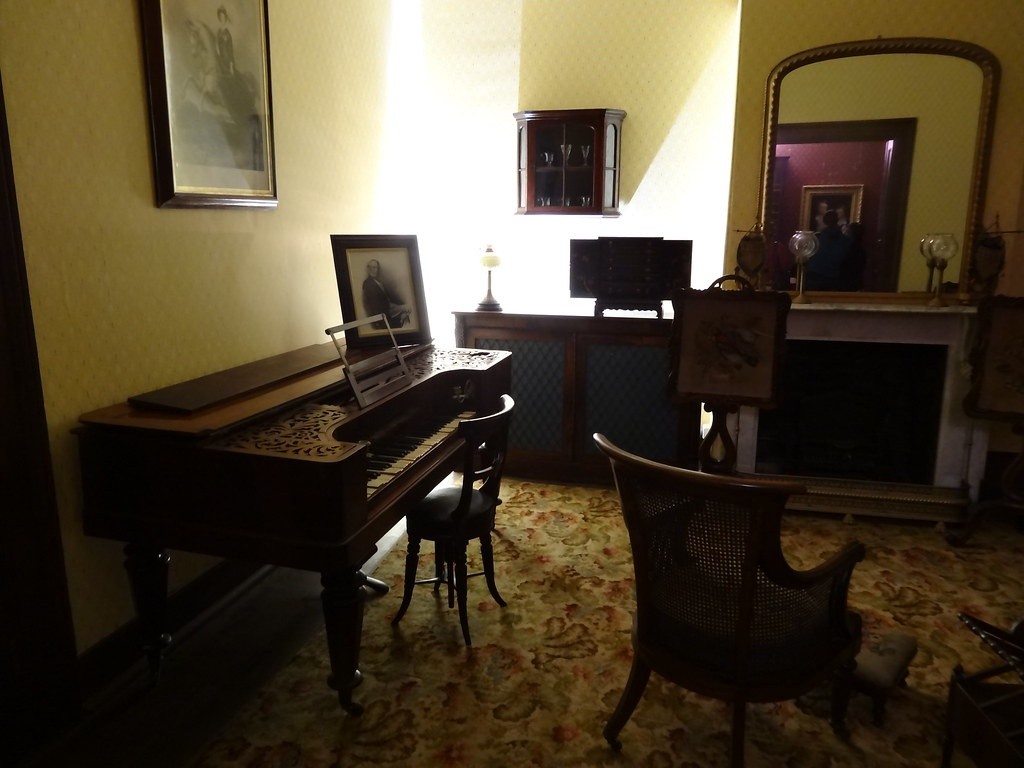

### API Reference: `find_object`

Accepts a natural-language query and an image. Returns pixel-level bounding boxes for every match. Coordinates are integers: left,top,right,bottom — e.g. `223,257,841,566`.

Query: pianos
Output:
69,338,516,718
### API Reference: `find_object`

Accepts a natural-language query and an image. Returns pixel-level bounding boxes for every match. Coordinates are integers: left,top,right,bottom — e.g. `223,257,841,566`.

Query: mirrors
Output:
754,37,1002,304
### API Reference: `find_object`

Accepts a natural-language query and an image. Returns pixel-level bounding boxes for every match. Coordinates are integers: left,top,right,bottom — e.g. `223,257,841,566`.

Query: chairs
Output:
389,391,517,644
940,610,1024,768
594,432,876,768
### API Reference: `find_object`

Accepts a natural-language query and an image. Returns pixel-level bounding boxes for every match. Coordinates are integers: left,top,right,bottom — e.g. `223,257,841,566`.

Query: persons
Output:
804,211,866,292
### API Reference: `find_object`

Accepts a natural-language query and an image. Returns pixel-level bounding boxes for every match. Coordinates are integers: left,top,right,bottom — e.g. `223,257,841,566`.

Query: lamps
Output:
478,245,504,310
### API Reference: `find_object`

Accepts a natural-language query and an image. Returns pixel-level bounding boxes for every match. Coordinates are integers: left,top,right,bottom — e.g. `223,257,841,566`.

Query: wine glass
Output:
581,145,591,165
561,144,572,166
546,151,554,168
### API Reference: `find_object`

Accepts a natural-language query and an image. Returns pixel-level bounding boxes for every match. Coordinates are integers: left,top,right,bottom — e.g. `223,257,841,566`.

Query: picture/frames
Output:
965,293,1024,424
135,1,282,210
674,288,788,409
800,185,864,235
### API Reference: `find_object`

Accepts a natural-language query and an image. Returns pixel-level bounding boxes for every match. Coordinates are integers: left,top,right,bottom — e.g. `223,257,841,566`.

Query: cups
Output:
540,196,550,205
562,197,571,206
581,197,590,206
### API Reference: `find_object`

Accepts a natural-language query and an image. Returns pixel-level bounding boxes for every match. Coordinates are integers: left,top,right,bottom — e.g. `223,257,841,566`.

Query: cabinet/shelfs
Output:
451,312,673,483
330,234,432,348
511,108,629,218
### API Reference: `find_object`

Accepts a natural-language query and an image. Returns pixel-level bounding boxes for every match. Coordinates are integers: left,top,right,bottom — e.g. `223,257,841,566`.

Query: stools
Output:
830,633,919,728
